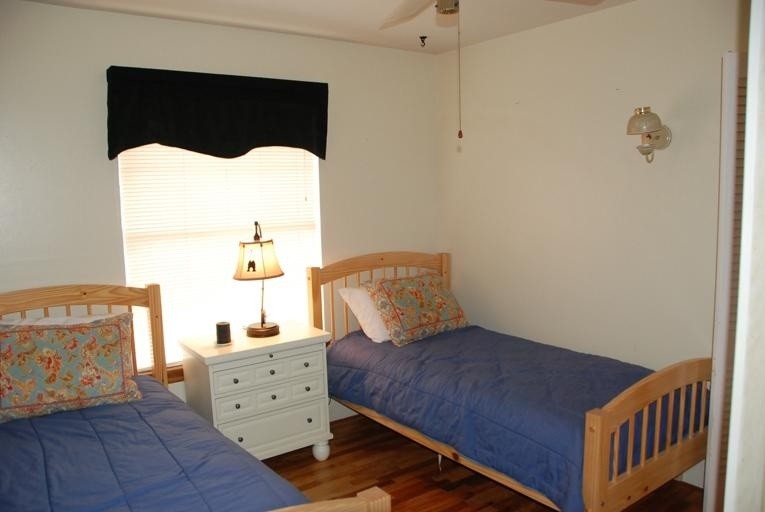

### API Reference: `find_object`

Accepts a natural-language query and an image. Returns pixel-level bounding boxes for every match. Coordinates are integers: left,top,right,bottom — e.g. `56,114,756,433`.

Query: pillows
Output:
338,274,475,348
0,310,146,422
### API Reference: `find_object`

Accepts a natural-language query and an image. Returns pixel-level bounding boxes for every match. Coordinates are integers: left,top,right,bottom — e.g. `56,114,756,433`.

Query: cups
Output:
217,323,231,343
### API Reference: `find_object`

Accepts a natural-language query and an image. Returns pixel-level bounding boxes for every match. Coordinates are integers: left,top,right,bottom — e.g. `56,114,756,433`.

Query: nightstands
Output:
176,319,334,461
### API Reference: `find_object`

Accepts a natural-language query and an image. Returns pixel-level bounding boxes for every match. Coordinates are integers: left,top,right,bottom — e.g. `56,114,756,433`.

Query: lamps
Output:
625,107,672,164
234,220,285,338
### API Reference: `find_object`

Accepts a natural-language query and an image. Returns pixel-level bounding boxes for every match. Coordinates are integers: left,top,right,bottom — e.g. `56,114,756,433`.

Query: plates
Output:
213,341,233,347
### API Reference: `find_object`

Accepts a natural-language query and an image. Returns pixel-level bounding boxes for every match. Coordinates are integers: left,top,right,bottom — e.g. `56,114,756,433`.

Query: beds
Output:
309,252,712,510
1,280,392,509
